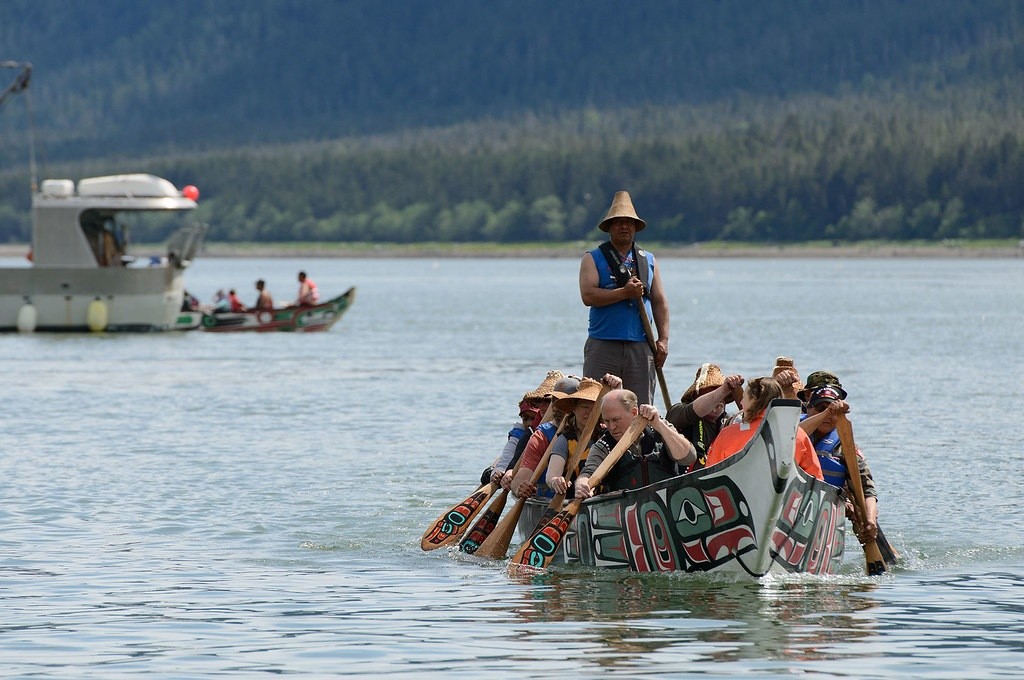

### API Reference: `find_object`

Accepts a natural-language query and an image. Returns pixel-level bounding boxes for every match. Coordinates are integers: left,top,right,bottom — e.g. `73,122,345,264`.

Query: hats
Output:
764,357,803,390
523,370,565,408
519,396,540,416
681,364,744,404
807,385,842,403
544,378,581,399
797,371,847,402
599,191,647,232
554,379,603,414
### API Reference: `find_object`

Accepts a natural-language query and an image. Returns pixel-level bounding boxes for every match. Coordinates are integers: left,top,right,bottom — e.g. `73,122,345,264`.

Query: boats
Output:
516,400,848,578
177,287,356,332
0,60,208,331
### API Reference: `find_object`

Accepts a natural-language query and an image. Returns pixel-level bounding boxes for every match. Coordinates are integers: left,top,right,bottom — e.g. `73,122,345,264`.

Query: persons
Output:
247,280,272,312
579,191,670,412
490,369,697,499
298,272,318,307
99,215,124,266
228,290,247,312
207,290,228,312
180,291,200,312
665,356,878,544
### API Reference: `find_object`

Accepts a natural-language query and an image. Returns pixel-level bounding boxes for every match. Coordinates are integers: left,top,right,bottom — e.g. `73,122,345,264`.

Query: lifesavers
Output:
257,307,275,326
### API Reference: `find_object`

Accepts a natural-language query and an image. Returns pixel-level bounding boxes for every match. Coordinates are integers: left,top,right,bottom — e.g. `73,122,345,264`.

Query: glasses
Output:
521,413,536,421
811,404,827,413
533,398,554,404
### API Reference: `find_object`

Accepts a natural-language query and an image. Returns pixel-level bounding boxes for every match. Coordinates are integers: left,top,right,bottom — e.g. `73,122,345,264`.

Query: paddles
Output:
630,277,673,413
525,377,617,544
830,401,901,577
472,411,577,560
508,413,650,586
420,476,499,552
457,399,562,555
